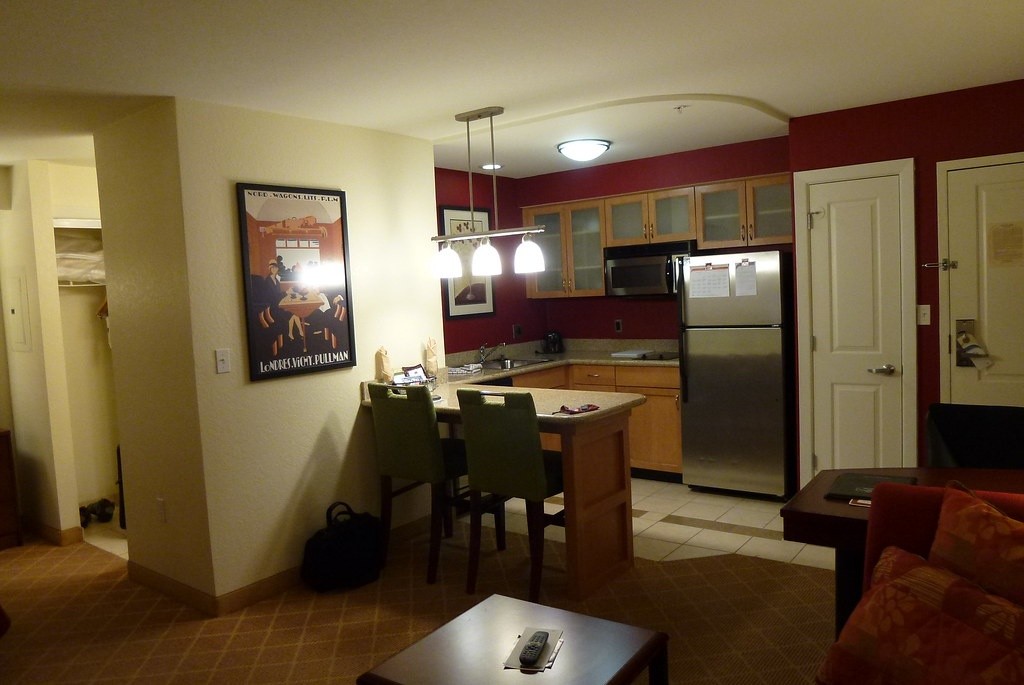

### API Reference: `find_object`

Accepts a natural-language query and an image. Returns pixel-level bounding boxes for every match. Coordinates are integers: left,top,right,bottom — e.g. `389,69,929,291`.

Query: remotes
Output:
519,631,549,665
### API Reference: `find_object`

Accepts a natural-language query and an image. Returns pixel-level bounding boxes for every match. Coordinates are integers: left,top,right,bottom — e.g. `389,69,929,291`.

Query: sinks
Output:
481,359,554,371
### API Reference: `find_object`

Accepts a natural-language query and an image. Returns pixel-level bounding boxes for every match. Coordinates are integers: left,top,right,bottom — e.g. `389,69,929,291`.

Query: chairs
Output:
457,388,575,601
926,403,1024,470
367,382,507,582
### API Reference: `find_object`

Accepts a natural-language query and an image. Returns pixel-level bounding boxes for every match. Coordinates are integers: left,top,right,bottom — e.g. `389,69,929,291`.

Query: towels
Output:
447,363,483,374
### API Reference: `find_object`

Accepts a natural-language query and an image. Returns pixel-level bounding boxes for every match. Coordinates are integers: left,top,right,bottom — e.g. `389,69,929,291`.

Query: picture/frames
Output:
439,205,496,318
236,181,358,380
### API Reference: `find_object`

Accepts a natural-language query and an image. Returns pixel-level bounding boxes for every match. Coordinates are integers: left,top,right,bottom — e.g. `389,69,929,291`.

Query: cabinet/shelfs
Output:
518,197,606,298
510,365,682,476
604,184,697,248
695,173,793,250
431,106,546,279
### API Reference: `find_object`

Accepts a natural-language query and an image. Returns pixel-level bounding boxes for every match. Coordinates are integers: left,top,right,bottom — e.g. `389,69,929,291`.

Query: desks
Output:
360,383,648,599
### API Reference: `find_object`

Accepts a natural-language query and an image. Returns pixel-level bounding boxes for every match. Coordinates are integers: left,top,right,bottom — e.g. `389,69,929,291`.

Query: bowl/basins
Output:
500,359,514,369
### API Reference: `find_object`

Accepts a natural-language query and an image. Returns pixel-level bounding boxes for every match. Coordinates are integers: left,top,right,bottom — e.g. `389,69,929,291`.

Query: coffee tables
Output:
356,594,670,685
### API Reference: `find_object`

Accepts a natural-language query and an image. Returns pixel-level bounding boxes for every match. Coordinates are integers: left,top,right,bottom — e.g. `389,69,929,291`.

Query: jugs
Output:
541,330,566,354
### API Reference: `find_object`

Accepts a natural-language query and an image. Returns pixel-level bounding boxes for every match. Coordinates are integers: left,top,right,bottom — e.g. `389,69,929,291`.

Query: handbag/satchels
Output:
303,501,383,589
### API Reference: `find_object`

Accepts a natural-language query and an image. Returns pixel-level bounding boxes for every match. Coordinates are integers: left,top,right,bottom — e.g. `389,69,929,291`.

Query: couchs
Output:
864,482,1024,599
780,470,1024,643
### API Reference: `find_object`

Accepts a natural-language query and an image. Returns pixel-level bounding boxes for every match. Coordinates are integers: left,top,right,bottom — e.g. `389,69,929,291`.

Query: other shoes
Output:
79,505,90,528
88,498,114,523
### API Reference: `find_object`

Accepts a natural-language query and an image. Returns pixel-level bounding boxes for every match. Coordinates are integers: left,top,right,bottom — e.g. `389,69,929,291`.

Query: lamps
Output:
556,140,612,162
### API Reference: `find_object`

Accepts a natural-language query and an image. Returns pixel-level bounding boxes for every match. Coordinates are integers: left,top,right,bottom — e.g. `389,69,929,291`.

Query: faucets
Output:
479,342,506,363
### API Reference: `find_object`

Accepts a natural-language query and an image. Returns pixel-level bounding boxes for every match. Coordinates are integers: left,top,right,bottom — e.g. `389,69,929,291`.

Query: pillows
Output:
817,548,1024,685
930,480,1024,605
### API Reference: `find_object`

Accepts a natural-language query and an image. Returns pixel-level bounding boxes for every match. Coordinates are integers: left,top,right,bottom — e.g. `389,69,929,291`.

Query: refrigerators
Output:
673,249,796,501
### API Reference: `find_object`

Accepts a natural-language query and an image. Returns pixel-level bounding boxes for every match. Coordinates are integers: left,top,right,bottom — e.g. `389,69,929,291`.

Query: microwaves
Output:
603,242,692,298
